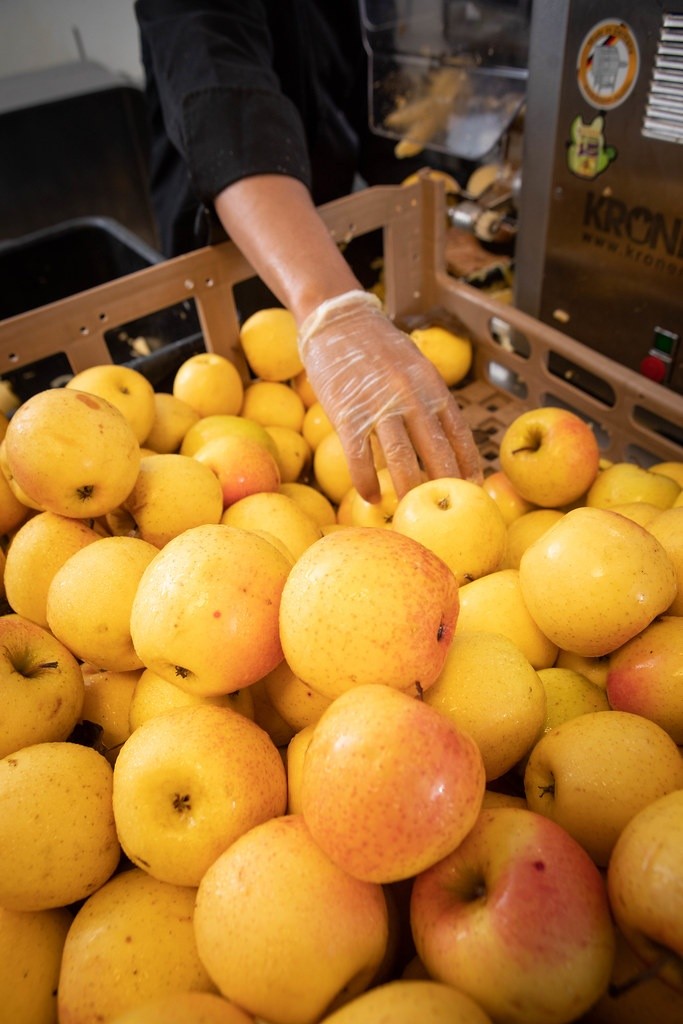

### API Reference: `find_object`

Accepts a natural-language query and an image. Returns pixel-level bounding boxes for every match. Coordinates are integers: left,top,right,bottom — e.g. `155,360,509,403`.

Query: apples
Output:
0,305,683,1024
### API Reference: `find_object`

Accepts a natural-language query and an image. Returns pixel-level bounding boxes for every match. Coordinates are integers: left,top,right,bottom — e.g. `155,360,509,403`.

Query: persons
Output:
133,1,484,504
